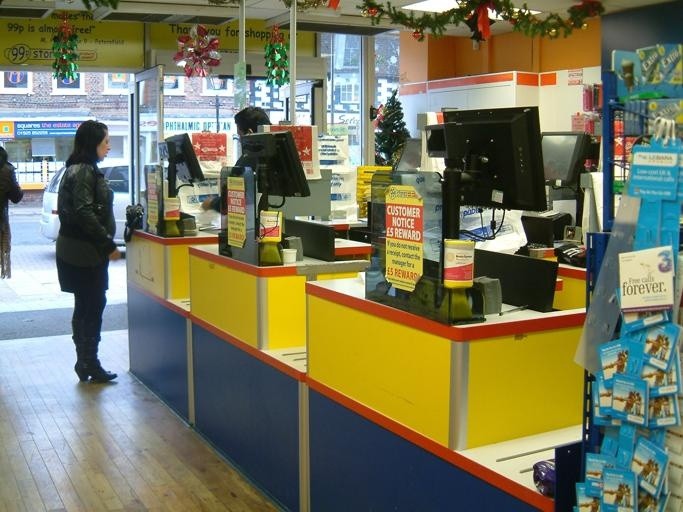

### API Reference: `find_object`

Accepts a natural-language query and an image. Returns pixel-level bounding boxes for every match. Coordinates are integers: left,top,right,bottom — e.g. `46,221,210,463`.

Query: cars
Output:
39,161,221,257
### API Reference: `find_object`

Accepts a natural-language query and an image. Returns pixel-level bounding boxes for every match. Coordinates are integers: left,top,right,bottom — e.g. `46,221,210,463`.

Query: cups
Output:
283,249,297,265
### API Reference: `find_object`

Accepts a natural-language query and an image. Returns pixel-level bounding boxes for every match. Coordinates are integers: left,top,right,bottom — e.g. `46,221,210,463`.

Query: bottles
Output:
366,257,384,293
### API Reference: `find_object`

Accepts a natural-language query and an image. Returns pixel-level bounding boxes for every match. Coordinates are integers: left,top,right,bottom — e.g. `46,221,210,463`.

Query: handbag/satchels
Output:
126,205,144,229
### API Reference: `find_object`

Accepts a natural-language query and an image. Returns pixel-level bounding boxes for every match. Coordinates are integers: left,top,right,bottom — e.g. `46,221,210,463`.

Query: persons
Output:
55,119,121,382
201,106,271,215
577,333,671,511
0,146,22,278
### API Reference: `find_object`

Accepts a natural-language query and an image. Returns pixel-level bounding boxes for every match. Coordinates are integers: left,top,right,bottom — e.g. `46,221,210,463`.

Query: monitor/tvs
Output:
541,132,587,186
444,106,547,211
165,134,205,184
241,131,311,197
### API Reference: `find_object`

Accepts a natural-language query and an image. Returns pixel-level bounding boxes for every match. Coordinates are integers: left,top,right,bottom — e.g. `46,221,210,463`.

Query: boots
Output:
72,336,118,381
